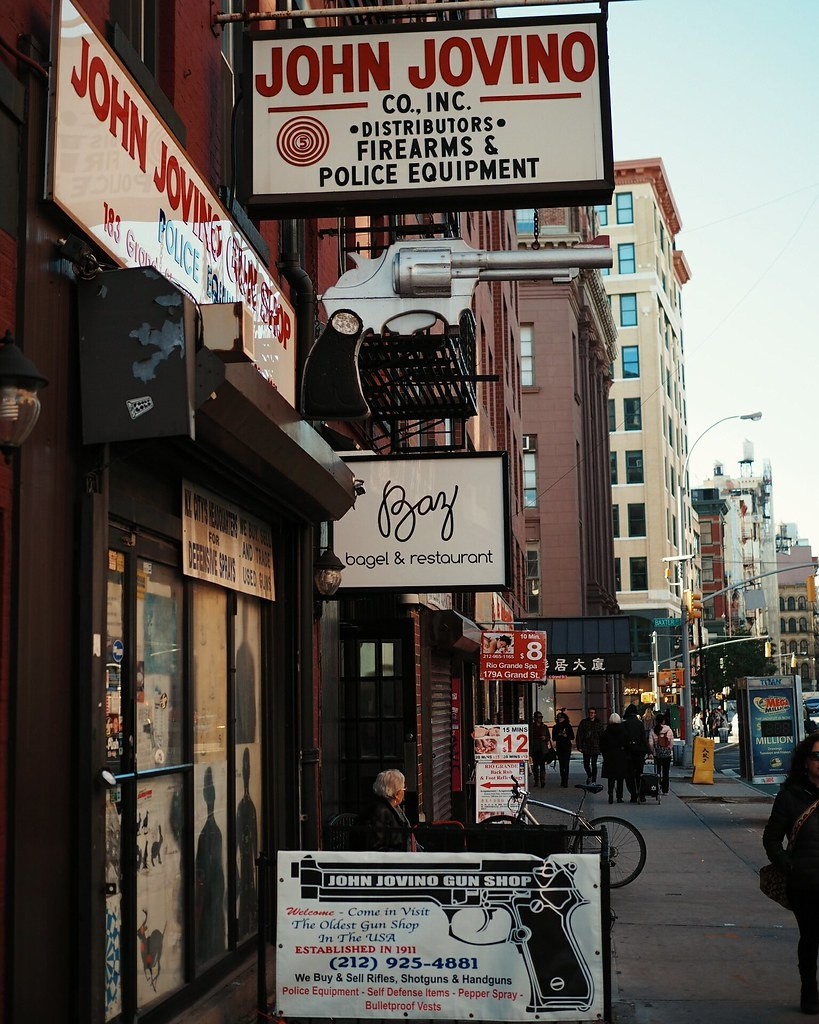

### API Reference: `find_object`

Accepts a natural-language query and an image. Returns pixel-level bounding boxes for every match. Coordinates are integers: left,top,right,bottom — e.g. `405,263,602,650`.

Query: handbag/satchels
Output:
558,728,572,746
544,740,556,764
760,864,792,911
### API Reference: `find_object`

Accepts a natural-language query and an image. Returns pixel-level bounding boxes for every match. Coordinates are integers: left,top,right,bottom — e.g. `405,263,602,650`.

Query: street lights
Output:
669,409,761,772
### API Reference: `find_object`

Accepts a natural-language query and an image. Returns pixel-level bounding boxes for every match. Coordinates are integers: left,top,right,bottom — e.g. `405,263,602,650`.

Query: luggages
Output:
637,757,663,805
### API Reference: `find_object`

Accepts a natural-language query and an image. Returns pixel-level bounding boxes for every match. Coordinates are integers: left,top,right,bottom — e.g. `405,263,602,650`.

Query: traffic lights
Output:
689,589,703,621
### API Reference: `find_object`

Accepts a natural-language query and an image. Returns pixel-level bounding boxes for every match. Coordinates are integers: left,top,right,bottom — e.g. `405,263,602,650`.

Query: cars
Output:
806,697,819,713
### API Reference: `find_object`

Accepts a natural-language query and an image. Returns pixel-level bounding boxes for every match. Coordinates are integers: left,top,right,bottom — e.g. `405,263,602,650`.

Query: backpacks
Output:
652,727,671,758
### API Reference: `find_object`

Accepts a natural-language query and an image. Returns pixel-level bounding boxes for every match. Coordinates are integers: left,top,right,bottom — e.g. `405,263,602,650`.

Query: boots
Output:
797,964,819,1015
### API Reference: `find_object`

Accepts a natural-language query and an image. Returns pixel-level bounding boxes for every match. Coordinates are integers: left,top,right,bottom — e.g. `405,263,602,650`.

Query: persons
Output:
763,734,819,1024
346,770,413,851
474,725,501,754
484,634,512,654
528,708,738,804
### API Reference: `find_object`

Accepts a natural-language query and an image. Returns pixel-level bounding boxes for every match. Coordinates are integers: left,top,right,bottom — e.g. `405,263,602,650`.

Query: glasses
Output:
809,751,819,761
538,716,543,718
589,712,595,714
558,716,563,719
402,786,408,791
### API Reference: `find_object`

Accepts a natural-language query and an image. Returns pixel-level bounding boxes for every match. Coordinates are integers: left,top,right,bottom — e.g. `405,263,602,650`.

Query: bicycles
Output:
477,775,647,889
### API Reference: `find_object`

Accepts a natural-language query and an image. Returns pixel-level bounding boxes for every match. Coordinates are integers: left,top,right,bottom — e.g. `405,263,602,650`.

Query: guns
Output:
297,239,615,421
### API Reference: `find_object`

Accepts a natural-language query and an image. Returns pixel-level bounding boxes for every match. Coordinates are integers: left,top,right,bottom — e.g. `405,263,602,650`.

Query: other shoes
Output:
534,783,539,787
560,777,568,788
630,797,637,802
592,776,596,784
617,799,624,803
586,778,591,785
609,793,613,804
540,783,545,788
